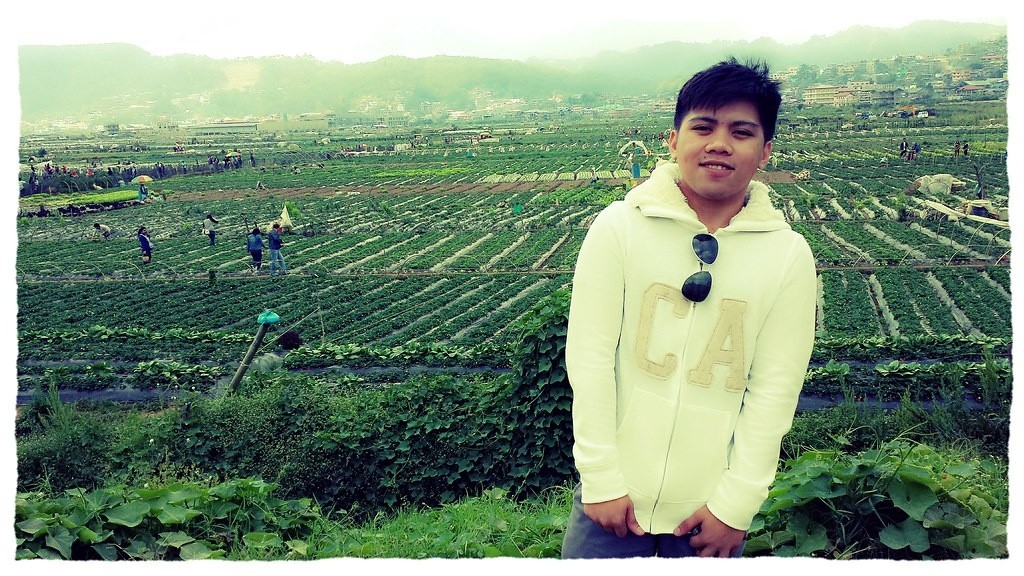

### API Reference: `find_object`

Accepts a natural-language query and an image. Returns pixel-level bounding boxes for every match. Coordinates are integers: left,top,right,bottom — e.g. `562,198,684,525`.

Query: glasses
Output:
682,232,718,308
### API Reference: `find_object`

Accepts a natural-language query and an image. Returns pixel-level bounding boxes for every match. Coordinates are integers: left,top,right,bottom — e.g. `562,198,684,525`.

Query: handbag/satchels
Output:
143,256,149,262
202,228,210,234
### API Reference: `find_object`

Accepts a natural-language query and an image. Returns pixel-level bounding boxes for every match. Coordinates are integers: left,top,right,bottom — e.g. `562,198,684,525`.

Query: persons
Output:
154,151,256,178
955,140,960,157
247,228,268,272
281,161,324,174
862,108,908,119
642,129,664,143
339,144,367,152
203,215,219,245
31,159,137,179
138,184,158,202
19,175,38,197
411,135,428,147
962,140,968,155
269,224,286,276
94,223,111,240
138,227,151,265
900,138,920,160
560,58,817,559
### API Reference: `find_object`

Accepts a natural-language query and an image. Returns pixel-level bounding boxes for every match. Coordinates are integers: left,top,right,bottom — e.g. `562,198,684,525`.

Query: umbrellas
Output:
226,152,241,167
131,175,153,199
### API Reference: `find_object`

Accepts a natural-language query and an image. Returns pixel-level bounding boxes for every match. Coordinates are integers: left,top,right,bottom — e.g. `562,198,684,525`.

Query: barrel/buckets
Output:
970,203,988,217
